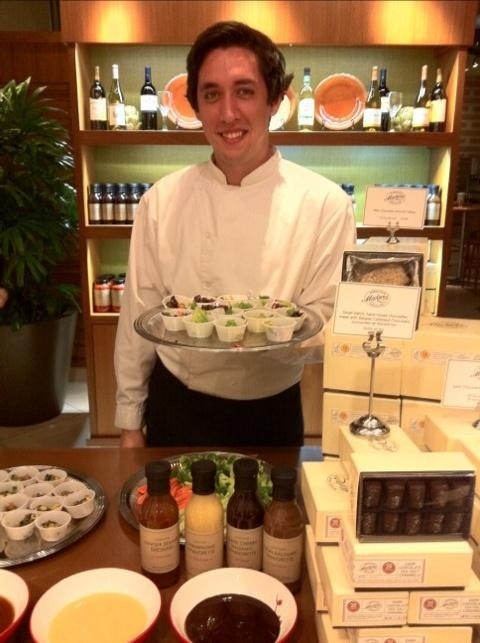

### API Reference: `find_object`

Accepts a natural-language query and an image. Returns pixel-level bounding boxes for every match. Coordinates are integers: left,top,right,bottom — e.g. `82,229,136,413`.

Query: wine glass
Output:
386,91,401,128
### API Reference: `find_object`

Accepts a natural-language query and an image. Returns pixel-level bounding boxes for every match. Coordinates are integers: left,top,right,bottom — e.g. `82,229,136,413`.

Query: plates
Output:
132,293,323,352
117,451,278,544
1,463,108,568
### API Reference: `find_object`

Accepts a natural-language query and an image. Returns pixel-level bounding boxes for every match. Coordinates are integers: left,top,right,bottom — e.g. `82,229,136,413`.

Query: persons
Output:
115,22,358,449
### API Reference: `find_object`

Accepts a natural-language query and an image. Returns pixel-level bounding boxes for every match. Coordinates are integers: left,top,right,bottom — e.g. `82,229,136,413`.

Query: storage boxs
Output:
297,312,479,637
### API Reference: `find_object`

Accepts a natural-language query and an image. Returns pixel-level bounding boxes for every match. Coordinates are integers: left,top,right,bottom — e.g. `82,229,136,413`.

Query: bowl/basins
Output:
182,315,214,338
243,310,276,335
249,296,271,307
193,294,217,306
35,511,72,543
190,303,215,312
37,467,67,483
31,464,53,473
218,293,248,304
214,306,243,317
0,569,29,643
313,73,368,129
25,483,54,507
161,294,190,309
164,72,203,127
28,567,162,641
193,304,227,318
212,318,248,342
52,479,87,497
271,87,298,129
275,307,307,331
167,566,297,643
160,309,191,331
29,496,62,512
0,469,8,482
266,301,296,311
263,317,297,342
2,509,35,541
0,496,28,528
64,489,96,520
0,480,24,500
10,465,38,482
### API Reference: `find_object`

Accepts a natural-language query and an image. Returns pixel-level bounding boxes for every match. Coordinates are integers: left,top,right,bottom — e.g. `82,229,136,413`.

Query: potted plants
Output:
0,75,79,433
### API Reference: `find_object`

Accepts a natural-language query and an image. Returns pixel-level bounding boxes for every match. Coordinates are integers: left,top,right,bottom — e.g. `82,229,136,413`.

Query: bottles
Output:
113,183,128,225
88,64,108,131
412,64,432,132
297,66,315,131
102,183,115,225
125,184,140,224
342,183,356,214
364,66,382,132
226,457,264,571
429,65,449,132
140,459,180,590
89,183,103,224
94,273,127,312
140,65,158,130
139,183,151,197
374,183,426,189
379,67,392,131
185,459,224,580
426,184,441,226
264,465,305,594
108,63,127,132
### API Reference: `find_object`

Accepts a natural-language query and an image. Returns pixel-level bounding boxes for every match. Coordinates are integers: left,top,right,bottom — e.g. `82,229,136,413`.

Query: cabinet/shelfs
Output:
59,0,480,438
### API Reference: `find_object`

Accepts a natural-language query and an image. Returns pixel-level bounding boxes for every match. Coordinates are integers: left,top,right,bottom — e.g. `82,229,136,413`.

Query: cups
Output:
156,89,174,130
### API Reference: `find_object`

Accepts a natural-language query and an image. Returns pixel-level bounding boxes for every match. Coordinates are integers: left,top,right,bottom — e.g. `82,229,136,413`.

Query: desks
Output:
0,446,323,643
